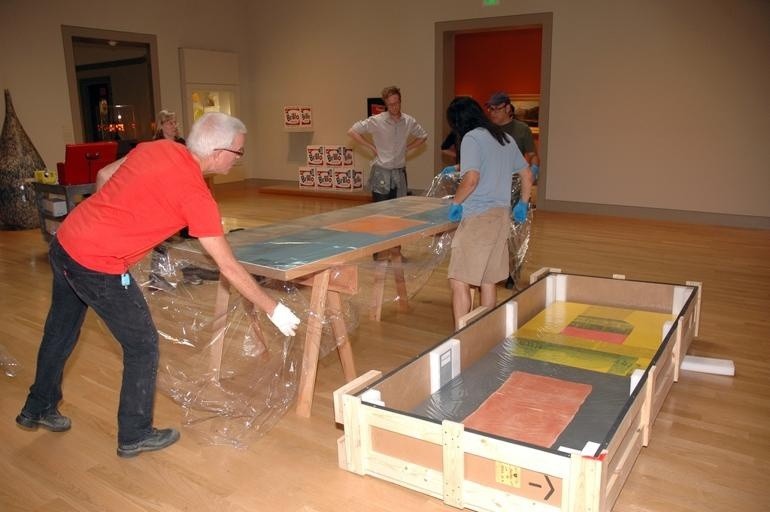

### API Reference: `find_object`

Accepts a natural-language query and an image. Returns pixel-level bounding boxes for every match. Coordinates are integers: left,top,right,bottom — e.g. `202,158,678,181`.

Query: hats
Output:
484,92,511,106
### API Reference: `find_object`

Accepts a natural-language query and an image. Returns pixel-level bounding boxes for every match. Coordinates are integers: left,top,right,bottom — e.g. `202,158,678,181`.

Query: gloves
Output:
530,164,539,185
266,301,300,337
448,202,463,223
512,199,528,224
441,165,456,175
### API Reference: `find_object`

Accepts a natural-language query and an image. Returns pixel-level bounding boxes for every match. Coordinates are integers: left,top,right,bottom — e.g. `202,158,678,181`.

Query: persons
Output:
440,90,542,290
148,110,204,291
441,127,464,165
14,113,302,458
440,94,533,332
345,84,429,263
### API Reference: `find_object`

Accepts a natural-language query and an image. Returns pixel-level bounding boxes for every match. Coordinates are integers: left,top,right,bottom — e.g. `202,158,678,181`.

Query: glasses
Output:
487,105,506,113
214,147,244,161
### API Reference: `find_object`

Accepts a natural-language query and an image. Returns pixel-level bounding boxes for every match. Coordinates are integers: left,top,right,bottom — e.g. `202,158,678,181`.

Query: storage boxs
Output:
57,141,118,185
299,145,363,191
285,106,312,125
41,199,68,235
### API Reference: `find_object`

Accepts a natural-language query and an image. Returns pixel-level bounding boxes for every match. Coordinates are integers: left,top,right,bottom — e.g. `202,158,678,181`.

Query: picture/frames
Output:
508,94,539,134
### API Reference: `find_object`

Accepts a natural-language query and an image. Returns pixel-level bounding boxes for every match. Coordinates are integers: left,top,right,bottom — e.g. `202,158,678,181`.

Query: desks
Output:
29,183,98,246
168,195,464,418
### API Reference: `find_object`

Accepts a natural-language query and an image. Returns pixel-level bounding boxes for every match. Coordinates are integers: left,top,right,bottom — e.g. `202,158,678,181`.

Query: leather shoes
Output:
116,427,181,459
15,409,72,432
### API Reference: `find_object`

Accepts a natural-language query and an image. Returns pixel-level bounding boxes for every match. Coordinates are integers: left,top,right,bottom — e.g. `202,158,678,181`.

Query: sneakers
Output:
183,275,203,285
505,274,520,289
149,278,177,292
